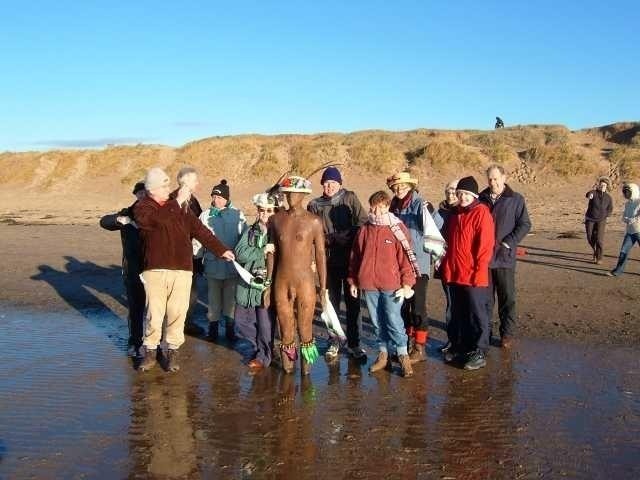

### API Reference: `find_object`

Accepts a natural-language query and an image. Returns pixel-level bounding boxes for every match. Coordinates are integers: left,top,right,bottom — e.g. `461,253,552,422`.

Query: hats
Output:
210,179,229,199
144,168,170,190
444,176,480,200
321,167,342,185
132,182,145,195
278,176,312,195
252,193,279,208
386,172,419,189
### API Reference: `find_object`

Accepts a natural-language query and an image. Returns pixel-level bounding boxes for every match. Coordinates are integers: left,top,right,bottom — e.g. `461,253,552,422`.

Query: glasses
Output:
260,209,272,214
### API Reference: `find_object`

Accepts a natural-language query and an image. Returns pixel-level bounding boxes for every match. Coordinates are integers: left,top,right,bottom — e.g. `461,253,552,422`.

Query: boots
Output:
226,322,237,339
368,352,392,372
139,349,157,370
398,337,426,378
166,349,180,372
205,320,218,342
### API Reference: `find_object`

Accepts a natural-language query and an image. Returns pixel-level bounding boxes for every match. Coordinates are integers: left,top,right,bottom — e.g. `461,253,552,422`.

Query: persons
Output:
99,167,248,373
438,179,459,352
385,171,448,365
307,167,369,358
605,182,640,278
584,179,613,266
347,191,416,379
438,176,496,371
262,175,328,375
233,192,285,369
479,164,531,349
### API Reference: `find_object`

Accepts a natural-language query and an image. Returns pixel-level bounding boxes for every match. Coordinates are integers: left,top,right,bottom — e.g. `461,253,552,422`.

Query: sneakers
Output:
185,323,204,334
348,346,367,360
442,342,487,371
501,336,513,348
326,343,338,358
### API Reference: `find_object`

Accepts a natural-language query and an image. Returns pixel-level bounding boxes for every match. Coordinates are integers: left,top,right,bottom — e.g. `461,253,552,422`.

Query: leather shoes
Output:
247,358,263,369
128,345,136,357
